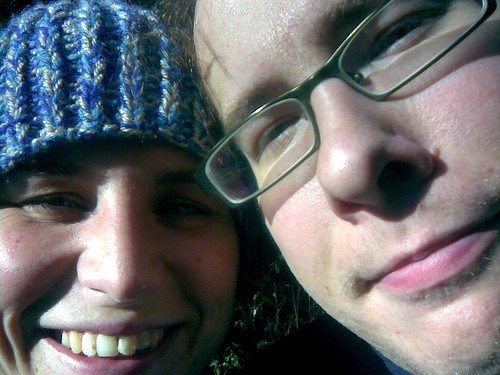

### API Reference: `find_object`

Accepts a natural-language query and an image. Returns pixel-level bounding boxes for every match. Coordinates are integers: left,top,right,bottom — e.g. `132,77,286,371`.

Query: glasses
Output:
192,0,500,208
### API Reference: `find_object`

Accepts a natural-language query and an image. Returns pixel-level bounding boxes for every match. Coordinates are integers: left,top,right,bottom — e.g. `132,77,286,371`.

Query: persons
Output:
160,0,500,375
0,0,246,375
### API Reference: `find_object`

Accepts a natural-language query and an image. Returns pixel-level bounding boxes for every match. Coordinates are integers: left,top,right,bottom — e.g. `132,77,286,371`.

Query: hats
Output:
0,0,241,178
